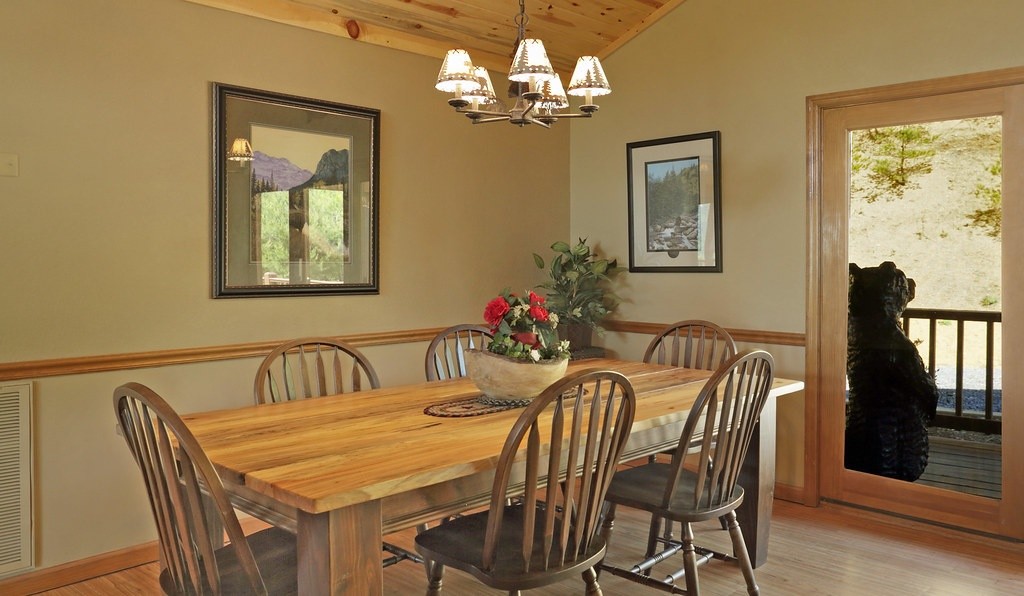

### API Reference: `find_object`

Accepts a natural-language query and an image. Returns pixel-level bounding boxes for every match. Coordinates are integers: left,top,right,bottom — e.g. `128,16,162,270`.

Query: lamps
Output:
434,0,613,129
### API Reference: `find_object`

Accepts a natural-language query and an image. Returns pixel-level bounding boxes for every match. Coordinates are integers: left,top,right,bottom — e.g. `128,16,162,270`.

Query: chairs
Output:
111,319,773,596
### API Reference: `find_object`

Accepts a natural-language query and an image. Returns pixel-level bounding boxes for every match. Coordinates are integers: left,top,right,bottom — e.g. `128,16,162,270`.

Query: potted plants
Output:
533,237,628,362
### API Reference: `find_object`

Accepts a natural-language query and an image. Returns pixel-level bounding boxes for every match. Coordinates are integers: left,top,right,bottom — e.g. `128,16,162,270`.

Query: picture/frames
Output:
625,130,723,274
210,81,381,299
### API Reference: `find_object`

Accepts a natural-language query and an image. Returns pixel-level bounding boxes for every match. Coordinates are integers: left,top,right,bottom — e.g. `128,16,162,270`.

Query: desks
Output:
117,357,805,596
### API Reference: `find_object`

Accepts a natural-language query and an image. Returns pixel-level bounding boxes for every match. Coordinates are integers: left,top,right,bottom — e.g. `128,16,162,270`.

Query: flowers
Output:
483,286,570,361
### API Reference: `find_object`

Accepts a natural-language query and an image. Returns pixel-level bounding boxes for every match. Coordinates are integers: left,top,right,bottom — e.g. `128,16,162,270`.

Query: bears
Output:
844,261,939,482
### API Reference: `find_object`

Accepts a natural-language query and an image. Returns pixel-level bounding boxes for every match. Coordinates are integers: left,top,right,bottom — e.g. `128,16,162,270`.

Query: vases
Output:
463,349,568,406
